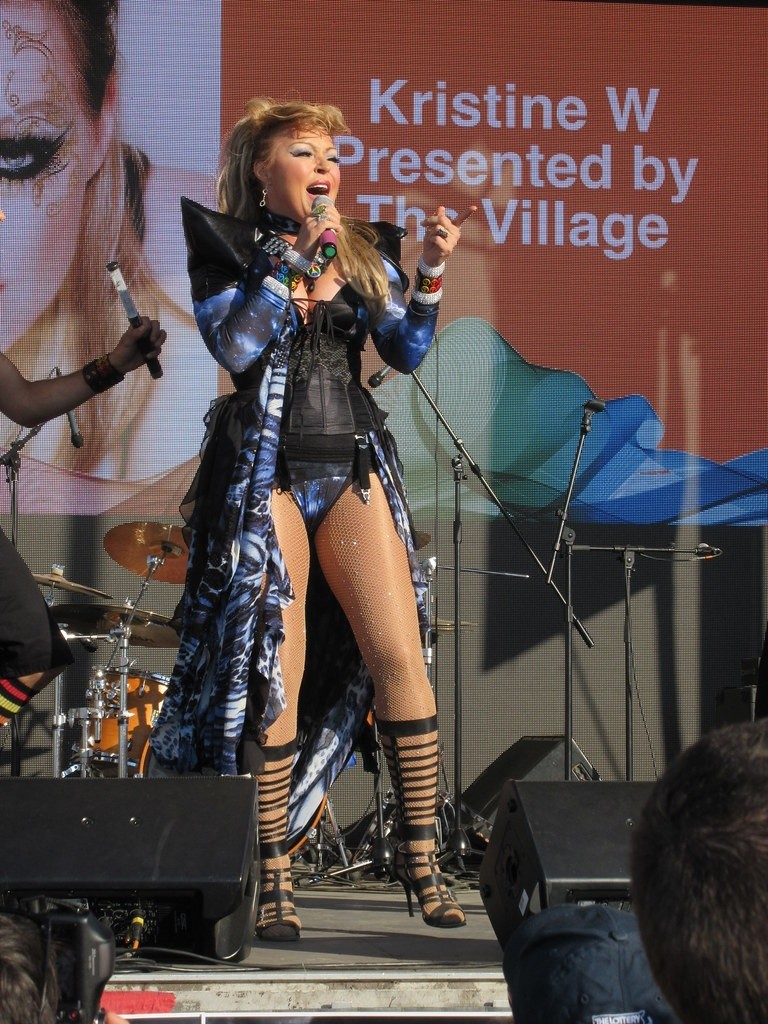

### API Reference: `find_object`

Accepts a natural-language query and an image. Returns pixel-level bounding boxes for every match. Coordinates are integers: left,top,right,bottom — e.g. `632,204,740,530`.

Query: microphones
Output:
695,542,722,556
311,196,337,259
367,365,392,388
56,366,85,448
73,632,98,652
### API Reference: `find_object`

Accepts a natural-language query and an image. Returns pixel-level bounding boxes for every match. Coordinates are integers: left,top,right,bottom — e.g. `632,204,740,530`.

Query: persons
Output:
0,909,128,1024
2,0,207,478
633,720,768,1024
0,318,167,743
150,97,478,941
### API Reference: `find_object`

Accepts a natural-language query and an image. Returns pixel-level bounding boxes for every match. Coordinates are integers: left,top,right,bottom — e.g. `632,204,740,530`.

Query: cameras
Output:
42,912,116,1024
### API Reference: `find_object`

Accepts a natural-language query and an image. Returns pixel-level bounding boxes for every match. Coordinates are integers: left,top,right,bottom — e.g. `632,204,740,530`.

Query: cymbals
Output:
30,571,115,602
430,616,478,633
415,530,432,551
48,601,182,649
101,520,192,585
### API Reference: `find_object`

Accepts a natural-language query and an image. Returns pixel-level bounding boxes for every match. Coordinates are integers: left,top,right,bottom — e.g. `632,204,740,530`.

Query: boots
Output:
374,713,466,923
255,737,302,940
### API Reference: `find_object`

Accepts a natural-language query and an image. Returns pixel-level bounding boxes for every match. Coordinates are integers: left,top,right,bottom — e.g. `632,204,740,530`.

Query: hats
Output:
503,903,678,1024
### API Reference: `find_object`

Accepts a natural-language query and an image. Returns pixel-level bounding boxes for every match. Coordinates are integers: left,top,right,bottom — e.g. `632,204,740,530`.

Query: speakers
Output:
479,780,659,952
446,735,601,849
0,777,263,964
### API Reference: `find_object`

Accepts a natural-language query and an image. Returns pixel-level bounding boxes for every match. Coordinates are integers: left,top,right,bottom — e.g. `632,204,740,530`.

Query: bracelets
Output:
411,252,443,305
270,248,312,292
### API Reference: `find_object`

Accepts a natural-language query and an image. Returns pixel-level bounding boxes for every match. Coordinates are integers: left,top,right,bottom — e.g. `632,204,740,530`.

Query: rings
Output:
317,212,331,222
310,205,326,216
436,229,448,239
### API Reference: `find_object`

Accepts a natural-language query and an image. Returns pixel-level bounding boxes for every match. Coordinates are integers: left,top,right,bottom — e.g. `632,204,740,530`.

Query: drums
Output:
136,723,330,862
73,664,172,777
